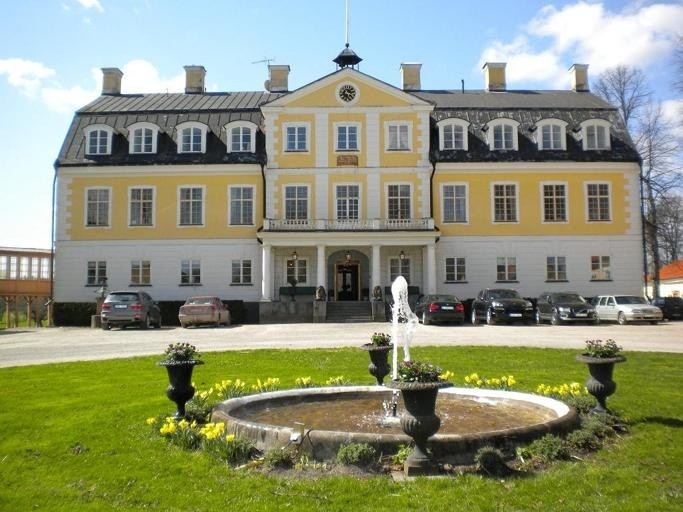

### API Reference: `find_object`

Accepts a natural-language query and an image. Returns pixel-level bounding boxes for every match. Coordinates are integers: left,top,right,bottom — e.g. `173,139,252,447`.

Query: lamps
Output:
398,251,406,261
344,251,352,267
288,252,298,266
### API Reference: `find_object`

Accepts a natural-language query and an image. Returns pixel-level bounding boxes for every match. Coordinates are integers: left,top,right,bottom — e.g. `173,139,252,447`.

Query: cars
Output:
100,290,162,330
413,288,683,325
178,294,232,328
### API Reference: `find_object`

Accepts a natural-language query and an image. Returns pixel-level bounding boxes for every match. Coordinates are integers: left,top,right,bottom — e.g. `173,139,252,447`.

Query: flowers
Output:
583,338,621,358
164,343,199,360
397,360,442,382
372,333,390,346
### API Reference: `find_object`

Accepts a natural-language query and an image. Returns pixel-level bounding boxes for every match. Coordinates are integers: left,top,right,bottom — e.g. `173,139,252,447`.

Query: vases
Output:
576,352,627,416
156,360,207,416
392,382,453,476
362,344,394,384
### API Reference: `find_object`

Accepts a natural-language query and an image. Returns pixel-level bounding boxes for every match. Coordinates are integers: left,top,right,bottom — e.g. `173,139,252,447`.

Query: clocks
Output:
336,83,357,104
